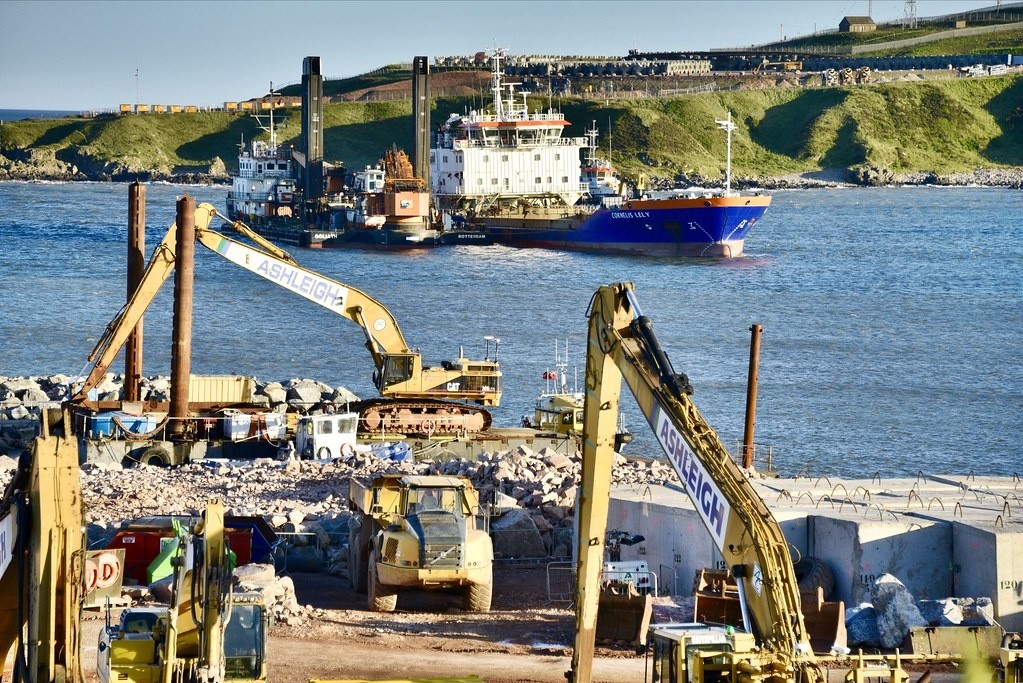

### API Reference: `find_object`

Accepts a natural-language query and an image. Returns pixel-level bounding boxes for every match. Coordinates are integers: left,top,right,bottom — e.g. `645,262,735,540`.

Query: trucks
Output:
348,464,495,615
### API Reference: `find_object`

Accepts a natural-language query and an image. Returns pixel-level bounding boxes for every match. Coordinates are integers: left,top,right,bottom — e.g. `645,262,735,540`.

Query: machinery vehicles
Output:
567,284,1023,683
70,203,506,435
0,407,267,683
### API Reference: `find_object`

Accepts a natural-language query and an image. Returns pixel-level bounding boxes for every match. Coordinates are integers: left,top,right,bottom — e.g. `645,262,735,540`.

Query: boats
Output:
522,338,636,454
429,34,773,256
225,81,493,248
